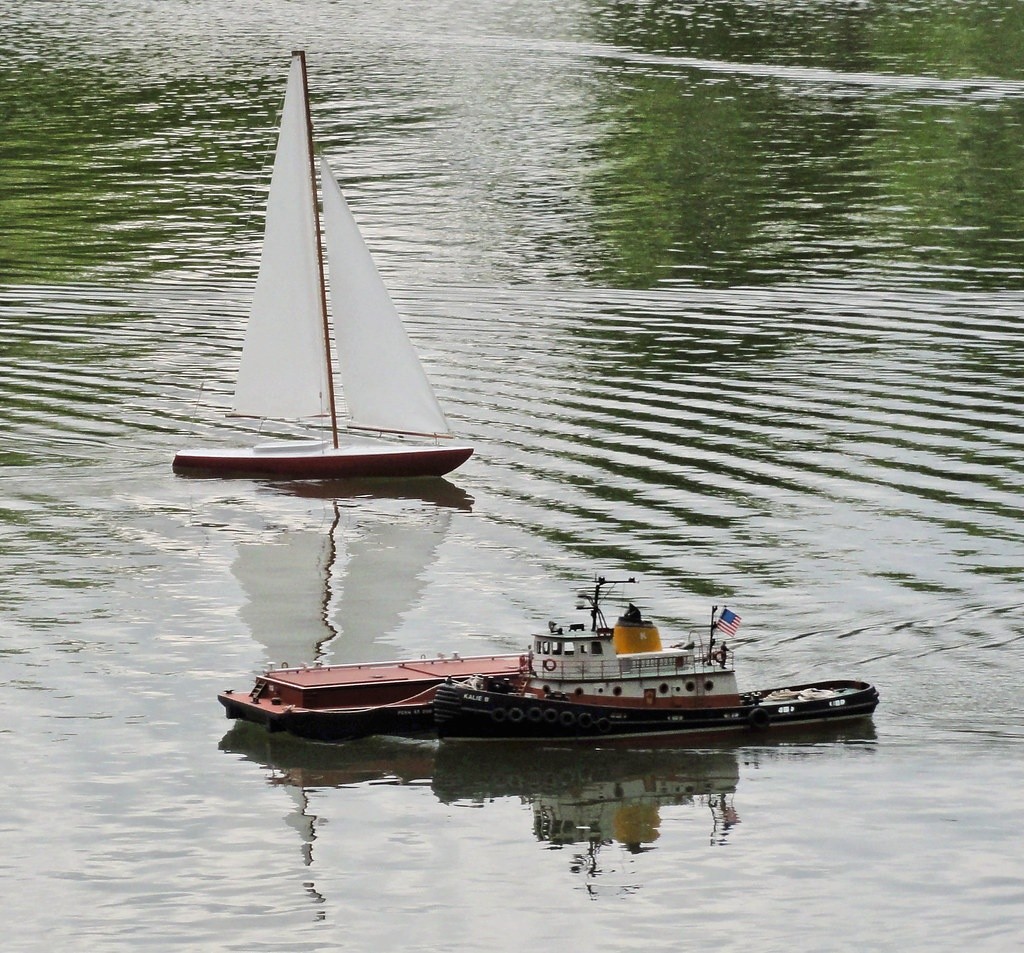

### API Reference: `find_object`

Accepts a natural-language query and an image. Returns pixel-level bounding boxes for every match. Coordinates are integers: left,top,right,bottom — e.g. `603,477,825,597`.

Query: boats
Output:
217,652,530,744
440,573,882,746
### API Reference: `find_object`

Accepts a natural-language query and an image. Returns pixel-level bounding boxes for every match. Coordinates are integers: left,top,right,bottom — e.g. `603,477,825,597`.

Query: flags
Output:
718,608,742,638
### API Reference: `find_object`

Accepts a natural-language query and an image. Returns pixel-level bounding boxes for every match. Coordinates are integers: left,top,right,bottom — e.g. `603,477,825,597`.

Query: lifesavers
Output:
544,659,557,671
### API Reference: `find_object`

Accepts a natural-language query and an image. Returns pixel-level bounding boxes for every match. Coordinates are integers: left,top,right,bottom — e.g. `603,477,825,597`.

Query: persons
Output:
719,641,734,670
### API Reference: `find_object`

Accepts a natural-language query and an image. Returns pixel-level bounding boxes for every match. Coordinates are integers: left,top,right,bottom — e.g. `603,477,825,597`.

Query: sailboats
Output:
168,46,475,480
